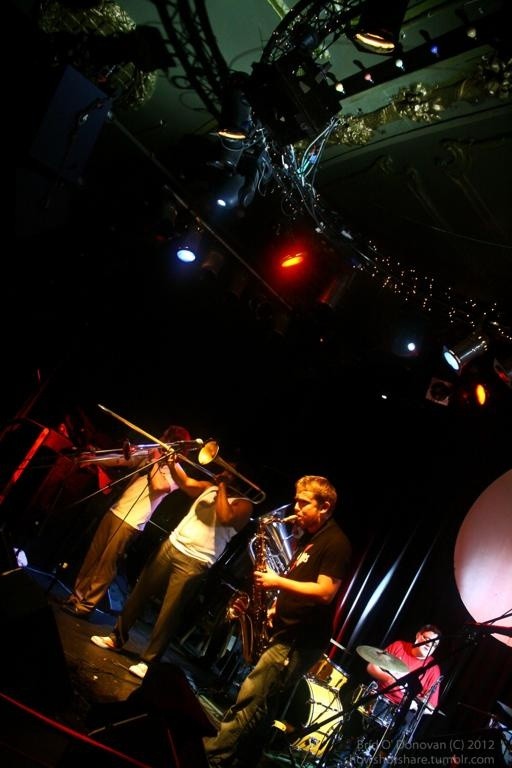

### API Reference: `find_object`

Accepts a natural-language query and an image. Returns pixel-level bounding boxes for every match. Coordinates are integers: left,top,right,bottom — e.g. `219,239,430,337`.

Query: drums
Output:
310,652,350,691
352,685,402,731
284,674,344,759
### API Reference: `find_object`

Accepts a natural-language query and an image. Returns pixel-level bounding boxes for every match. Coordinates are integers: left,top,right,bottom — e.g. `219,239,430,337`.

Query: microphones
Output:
176,438,204,449
427,675,444,692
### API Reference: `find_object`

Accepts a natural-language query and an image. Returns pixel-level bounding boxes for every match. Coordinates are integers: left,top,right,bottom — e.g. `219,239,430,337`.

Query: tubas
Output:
245,503,291,604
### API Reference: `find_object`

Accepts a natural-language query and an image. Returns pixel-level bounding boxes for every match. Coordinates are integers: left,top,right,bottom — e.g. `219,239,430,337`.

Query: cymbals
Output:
331,639,354,658
356,645,408,676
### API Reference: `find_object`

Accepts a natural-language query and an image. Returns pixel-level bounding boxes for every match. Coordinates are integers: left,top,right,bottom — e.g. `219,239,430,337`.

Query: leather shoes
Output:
55,593,75,605
64,603,92,623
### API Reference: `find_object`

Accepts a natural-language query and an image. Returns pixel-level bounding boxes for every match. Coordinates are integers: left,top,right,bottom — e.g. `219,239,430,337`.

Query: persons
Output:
60,423,203,617
323,621,446,767
202,473,356,754
87,455,255,680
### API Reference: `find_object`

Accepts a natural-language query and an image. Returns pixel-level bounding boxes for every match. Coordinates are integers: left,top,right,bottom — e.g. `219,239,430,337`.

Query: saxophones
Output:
227,513,299,664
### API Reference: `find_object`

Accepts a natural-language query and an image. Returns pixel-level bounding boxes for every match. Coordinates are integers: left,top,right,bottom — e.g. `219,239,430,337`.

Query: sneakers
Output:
92,635,123,653
129,662,147,678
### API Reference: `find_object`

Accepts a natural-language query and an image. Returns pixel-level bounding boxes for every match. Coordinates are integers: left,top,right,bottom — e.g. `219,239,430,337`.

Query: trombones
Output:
74,439,203,465
98,404,267,505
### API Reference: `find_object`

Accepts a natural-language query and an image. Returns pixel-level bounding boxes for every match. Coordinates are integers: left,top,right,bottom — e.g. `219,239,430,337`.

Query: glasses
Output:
423,635,437,647
